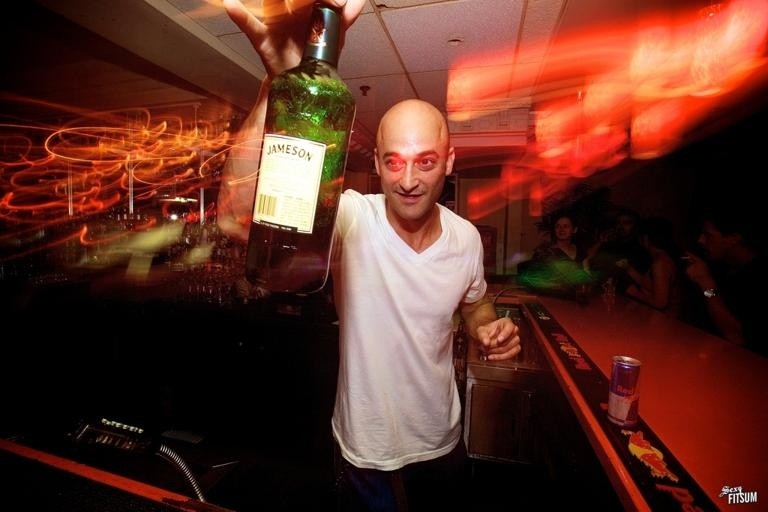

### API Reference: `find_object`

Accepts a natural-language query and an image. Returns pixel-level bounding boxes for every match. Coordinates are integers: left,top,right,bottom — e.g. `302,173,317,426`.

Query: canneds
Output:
606,354,642,427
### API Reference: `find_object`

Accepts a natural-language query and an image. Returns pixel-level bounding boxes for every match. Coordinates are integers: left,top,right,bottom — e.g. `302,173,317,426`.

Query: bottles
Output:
0,208,238,306
245,0,357,300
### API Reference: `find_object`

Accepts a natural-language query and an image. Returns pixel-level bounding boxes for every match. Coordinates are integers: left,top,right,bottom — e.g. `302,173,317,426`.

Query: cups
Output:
574,282,592,308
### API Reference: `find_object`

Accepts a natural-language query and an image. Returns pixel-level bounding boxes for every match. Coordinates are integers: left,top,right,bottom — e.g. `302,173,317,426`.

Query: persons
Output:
216,0,522,512
534,202,767,360
